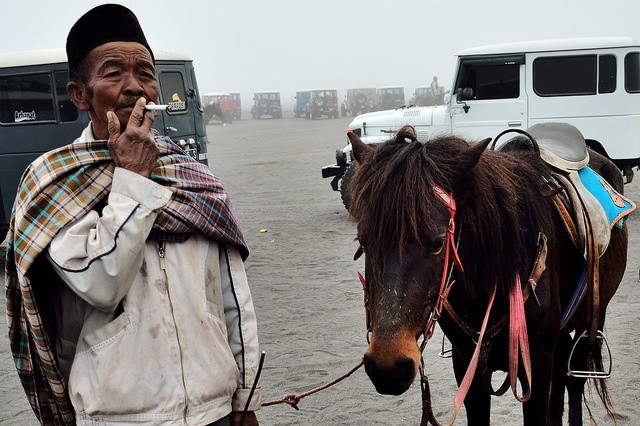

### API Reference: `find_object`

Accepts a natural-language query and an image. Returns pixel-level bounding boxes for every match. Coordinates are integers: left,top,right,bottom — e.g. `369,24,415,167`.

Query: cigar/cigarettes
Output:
145,105,170,111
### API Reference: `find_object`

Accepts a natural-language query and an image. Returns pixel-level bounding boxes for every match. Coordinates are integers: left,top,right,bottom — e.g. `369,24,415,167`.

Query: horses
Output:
346,128,631,426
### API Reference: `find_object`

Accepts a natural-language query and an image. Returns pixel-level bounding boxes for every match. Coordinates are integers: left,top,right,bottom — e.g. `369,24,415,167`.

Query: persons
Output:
0,3,261,426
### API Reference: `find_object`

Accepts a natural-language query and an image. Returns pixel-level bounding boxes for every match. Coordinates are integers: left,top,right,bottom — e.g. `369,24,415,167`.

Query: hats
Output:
66,3,154,77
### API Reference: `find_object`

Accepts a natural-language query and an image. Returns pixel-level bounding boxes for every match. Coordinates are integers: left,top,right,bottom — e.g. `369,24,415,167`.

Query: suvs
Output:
305,89,338,119
377,87,405,111
202,92,243,121
341,88,377,116
408,86,444,107
318,35,640,221
294,91,310,117
252,92,282,121
1,59,209,242
